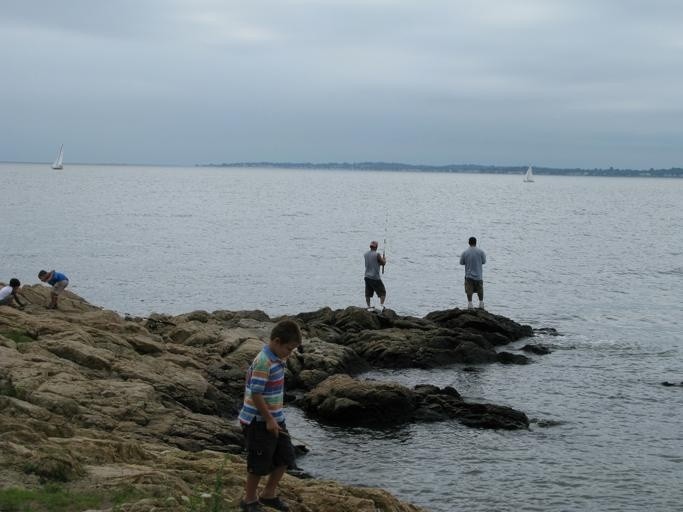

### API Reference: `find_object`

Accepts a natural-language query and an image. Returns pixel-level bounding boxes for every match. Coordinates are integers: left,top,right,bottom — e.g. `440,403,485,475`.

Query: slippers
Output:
240,496,265,511
257,492,290,512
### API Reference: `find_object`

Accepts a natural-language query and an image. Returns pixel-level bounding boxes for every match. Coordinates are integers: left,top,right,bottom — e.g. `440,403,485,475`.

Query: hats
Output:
369,241,378,248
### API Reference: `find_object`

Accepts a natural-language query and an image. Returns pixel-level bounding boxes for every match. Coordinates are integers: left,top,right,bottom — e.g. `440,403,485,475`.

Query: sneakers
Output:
467,301,485,310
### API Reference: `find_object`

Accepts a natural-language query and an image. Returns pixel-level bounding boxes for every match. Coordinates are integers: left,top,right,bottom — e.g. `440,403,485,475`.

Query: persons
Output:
362,241,386,310
36,269,70,310
234,316,304,512
459,236,487,310
0,277,28,312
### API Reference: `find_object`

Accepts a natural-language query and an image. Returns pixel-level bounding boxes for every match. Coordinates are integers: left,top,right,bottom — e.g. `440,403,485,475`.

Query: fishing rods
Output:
382,212,387,273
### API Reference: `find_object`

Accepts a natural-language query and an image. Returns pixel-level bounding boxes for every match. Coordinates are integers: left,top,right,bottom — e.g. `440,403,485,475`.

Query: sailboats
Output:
52,145,64,169
523,165,534,182
